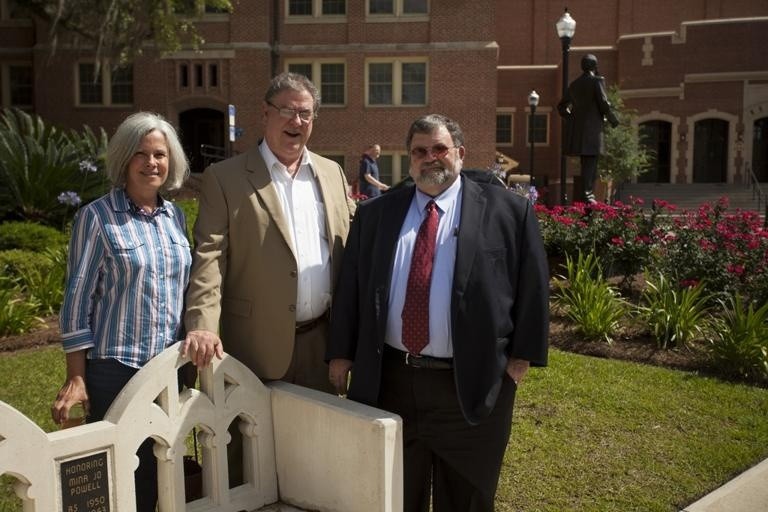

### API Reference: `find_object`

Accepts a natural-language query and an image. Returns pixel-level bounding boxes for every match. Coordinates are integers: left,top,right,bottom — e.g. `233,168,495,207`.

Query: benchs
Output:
0,338,404,512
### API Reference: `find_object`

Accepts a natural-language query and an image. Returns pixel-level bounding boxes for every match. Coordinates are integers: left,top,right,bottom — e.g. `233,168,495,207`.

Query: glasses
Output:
265,98,315,120
407,145,460,158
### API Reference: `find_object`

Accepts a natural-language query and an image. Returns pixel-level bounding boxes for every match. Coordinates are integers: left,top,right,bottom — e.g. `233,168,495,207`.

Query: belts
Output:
295,308,333,336
383,345,455,372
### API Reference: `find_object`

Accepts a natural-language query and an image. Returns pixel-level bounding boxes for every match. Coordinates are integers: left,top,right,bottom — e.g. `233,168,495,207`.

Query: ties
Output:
400,200,440,355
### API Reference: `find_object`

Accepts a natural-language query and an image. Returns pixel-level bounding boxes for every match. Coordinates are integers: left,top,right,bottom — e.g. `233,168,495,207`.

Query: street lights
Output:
525,90,543,180
555,4,576,207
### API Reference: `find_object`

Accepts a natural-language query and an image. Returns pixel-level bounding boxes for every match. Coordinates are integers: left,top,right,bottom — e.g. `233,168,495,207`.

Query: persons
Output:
359,143,391,197
182,72,350,486
327,114,549,512
50,112,194,512
556,53,619,204
